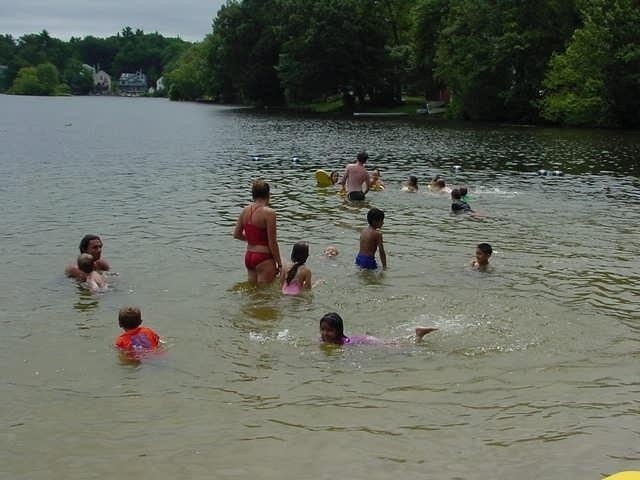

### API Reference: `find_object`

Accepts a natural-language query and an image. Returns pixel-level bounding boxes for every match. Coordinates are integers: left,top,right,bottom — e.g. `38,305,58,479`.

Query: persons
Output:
434,179,453,193
319,311,440,355
234,182,281,285
281,241,312,296
459,187,474,202
331,170,339,183
323,245,339,257
450,188,472,215
402,175,419,193
470,243,493,272
65,234,109,276
356,207,387,270
342,150,369,203
369,168,382,190
78,251,106,292
116,305,168,363
